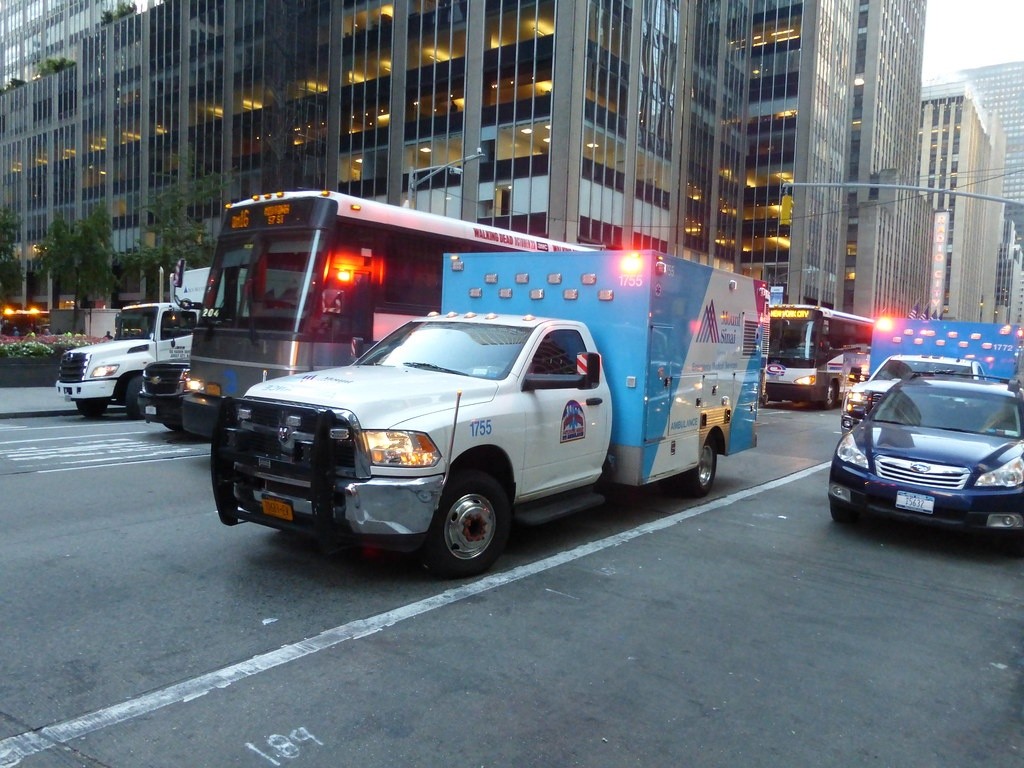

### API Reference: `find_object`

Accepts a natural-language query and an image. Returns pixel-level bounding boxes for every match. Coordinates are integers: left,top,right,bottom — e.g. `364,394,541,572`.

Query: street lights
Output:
409,154,486,211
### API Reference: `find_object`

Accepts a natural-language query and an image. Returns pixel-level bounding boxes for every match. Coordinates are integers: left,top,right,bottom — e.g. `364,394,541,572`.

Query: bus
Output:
181,191,605,438
760,304,875,410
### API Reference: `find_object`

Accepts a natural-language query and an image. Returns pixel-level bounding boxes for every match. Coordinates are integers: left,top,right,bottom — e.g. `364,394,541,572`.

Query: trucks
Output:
54,267,316,421
209,249,772,581
1,307,121,341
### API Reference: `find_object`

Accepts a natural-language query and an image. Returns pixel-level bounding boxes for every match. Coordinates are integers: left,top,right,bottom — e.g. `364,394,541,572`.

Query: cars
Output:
136,355,188,430
840,354,987,437
828,368,1024,556
